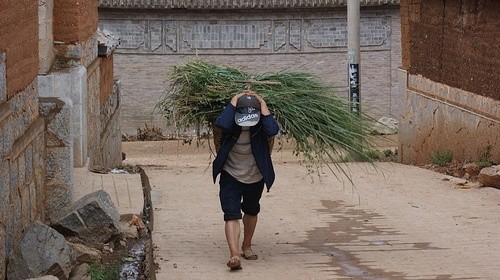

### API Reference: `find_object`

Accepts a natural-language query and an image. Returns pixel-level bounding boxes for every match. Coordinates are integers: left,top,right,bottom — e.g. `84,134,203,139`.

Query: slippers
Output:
241,251,259,260
227,256,240,268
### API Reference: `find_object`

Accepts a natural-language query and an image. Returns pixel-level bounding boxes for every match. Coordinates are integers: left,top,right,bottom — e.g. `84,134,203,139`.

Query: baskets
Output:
212,111,274,156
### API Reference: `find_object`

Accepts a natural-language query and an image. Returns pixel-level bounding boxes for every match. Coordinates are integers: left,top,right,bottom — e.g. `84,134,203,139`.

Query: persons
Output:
212,91,280,270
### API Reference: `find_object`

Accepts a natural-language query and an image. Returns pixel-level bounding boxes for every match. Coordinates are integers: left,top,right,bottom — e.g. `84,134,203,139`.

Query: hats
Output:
235,95,260,126
